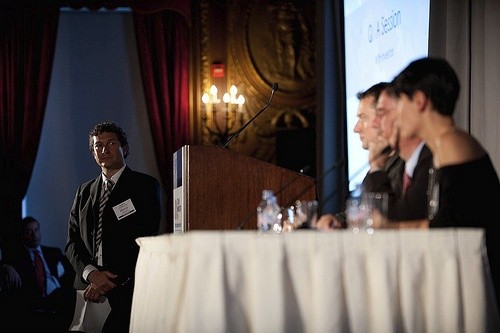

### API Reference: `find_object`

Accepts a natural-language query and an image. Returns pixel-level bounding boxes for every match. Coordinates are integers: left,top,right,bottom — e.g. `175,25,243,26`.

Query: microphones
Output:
223,82,279,148
237,145,392,230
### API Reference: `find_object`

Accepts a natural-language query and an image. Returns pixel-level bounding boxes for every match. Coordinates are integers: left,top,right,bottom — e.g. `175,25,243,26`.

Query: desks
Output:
130,226,491,333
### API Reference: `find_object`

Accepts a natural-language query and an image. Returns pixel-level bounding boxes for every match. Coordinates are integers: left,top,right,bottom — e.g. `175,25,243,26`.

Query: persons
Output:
284,82,390,229
316,84,433,231
0,217,65,333
387,57,500,289
64,121,163,333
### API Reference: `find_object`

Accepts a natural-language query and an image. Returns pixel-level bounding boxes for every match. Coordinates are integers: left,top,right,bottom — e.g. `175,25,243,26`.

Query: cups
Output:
296,201,318,229
343,192,389,233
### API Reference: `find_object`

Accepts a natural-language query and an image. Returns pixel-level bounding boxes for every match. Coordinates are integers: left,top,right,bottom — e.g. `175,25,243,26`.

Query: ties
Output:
403,170,411,191
94,180,115,253
33,250,48,293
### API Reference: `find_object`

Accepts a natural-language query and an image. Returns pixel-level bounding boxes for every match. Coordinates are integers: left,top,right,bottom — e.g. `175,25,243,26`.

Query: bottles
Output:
257,189,282,232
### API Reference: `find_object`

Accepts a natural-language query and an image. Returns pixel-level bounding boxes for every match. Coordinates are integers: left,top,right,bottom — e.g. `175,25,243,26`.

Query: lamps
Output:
201,84,248,149
